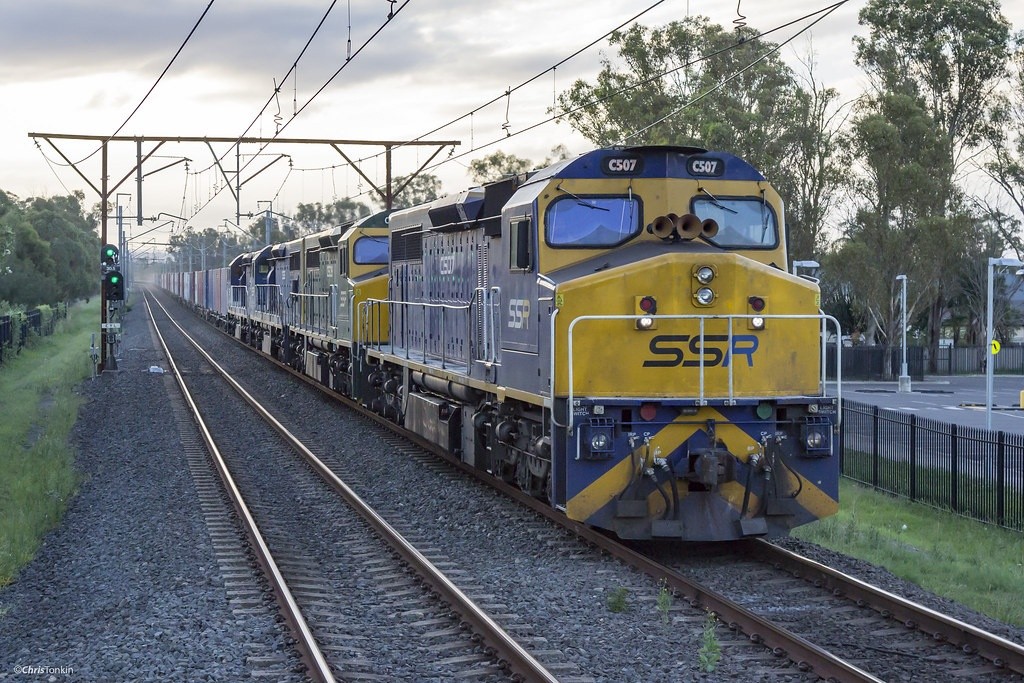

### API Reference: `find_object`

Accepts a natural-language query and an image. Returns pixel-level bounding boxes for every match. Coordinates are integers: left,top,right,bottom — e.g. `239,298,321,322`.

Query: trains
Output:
230,146,842,542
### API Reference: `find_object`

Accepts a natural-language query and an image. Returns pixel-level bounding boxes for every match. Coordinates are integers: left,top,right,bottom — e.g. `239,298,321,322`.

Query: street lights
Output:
896,275,907,377
986,257,1024,430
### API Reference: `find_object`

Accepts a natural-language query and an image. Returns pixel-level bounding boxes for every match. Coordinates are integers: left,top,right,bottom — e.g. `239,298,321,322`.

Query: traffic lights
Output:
101,244,118,264
105,270,124,300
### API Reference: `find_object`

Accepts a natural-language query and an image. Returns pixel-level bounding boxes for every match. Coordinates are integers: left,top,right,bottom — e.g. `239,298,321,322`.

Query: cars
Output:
1009,336,1024,346
820,326,866,347
